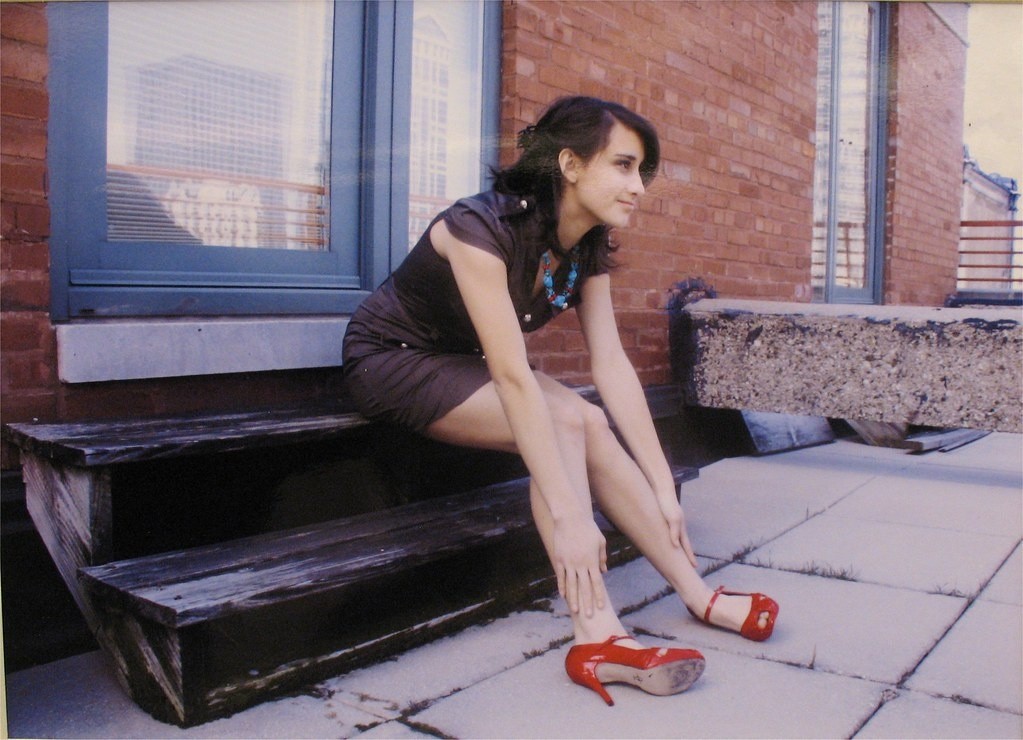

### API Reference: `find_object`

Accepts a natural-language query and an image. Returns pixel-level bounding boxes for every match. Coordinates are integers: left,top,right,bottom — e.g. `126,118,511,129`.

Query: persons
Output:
341,96,779,705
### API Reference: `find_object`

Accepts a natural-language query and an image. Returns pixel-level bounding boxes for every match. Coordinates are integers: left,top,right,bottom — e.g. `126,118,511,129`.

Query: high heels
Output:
565,635,708,706
688,586,779,641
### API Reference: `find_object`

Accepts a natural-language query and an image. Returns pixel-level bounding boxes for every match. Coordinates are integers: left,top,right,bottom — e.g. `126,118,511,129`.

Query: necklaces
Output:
542,245,580,310
